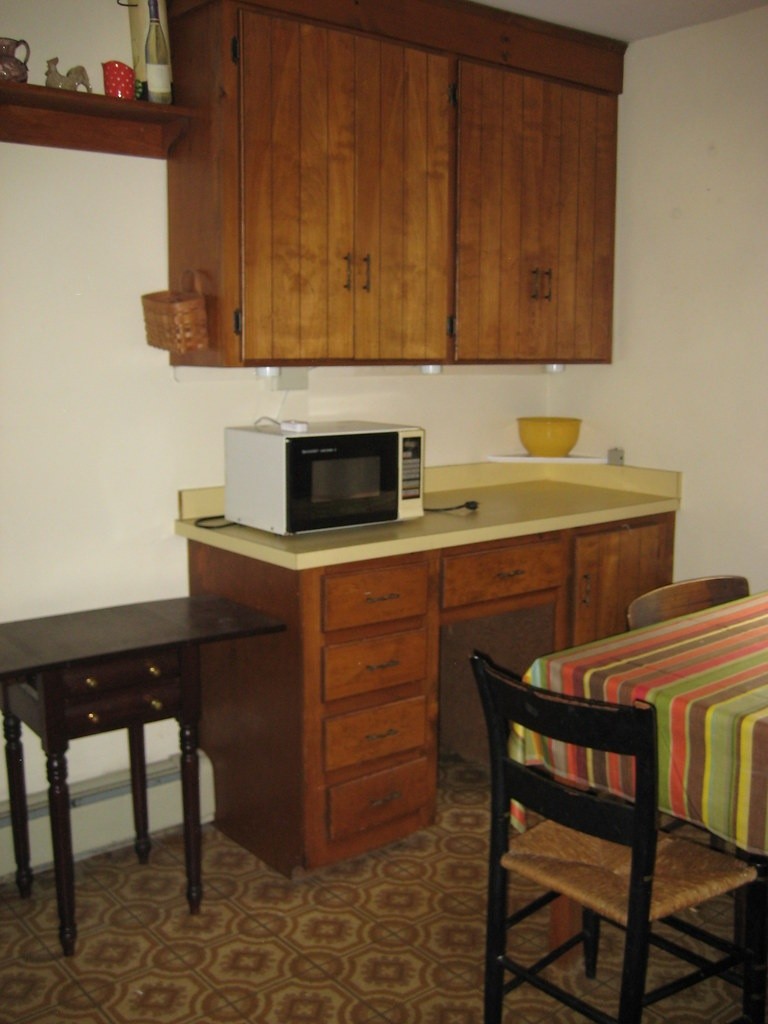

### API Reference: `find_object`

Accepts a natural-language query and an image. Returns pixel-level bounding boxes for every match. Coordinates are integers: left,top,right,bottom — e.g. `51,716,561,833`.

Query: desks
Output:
508,591,767,1024
0,593,288,958
175,458,684,879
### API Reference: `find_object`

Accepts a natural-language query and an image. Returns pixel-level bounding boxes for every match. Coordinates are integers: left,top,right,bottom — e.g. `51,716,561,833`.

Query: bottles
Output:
143,0,172,105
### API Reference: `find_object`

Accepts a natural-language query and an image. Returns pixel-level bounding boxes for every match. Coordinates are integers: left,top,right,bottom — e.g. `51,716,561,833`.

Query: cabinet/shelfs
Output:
168,0,615,368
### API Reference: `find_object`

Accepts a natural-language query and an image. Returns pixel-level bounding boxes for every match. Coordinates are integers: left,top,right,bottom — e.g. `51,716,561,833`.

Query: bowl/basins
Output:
514,417,582,458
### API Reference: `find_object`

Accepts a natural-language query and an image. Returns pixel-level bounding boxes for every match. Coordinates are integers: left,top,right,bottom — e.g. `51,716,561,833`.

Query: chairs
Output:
467,647,759,1023
625,574,749,630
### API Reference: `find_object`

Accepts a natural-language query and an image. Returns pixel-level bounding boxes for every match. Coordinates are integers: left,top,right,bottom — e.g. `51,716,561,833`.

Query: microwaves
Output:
219,420,426,539
